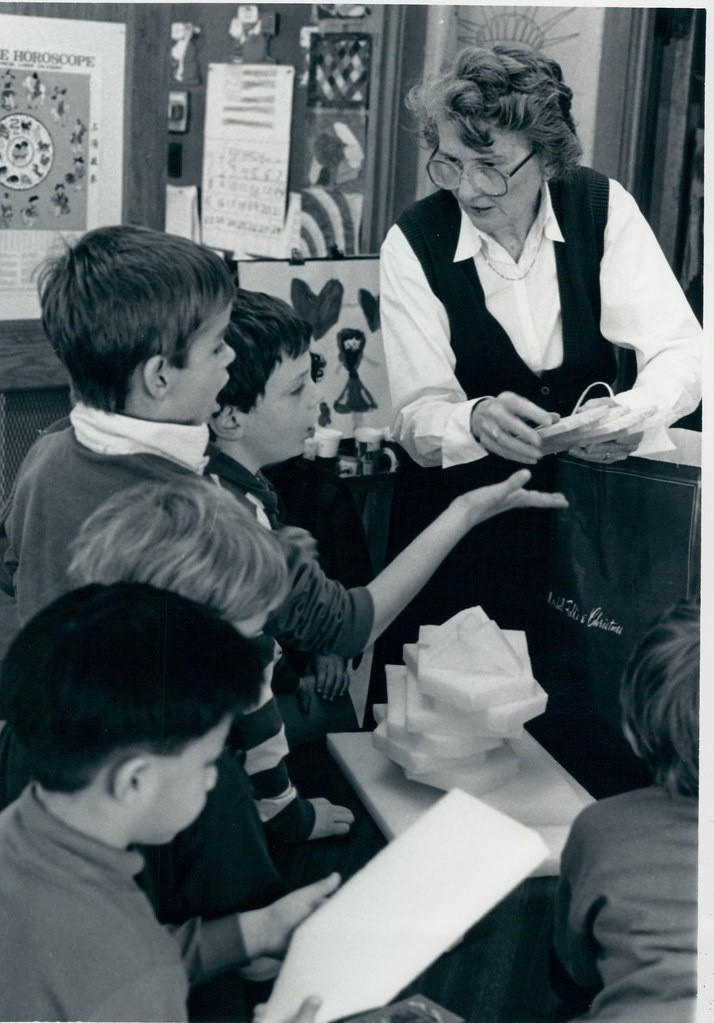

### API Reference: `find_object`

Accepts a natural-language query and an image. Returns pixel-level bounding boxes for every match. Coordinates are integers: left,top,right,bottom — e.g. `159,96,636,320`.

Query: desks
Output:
326,729,600,882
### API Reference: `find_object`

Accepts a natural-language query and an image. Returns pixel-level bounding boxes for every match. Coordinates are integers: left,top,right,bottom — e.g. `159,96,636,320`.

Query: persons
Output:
0,225,700,1023
362,43,704,730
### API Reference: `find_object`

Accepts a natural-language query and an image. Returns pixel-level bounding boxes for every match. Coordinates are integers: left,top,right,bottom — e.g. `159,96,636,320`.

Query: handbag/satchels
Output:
492,380,700,776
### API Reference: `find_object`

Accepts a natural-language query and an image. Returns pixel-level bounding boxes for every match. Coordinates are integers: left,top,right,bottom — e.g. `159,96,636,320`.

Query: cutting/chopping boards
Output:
253,787,549,1023
324,732,599,877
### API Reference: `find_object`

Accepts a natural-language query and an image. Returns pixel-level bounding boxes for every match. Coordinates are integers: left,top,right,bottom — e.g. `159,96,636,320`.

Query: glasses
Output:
426,146,537,196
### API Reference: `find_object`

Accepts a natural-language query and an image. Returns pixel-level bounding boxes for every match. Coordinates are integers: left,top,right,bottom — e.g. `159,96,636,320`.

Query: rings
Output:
490,429,498,441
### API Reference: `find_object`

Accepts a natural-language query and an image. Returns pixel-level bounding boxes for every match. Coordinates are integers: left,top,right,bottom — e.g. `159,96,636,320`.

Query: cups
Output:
315,428,342,458
355,427,384,475
